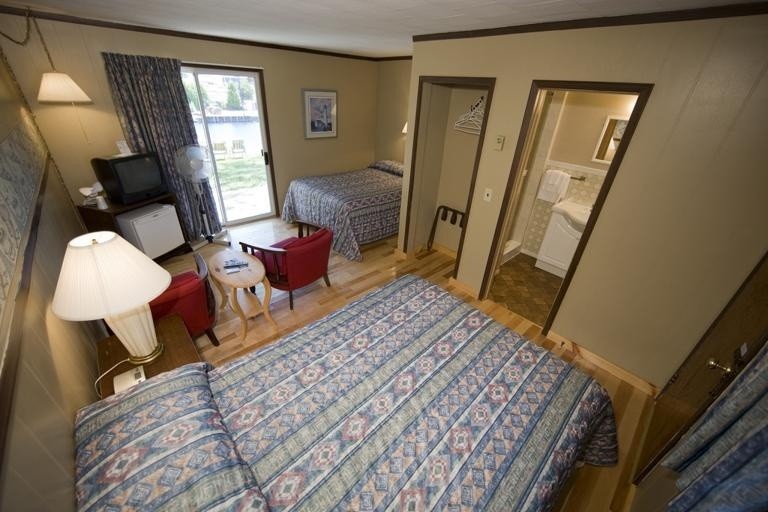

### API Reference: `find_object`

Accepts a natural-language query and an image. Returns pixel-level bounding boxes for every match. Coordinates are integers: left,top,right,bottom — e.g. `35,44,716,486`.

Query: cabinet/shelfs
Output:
80,196,191,261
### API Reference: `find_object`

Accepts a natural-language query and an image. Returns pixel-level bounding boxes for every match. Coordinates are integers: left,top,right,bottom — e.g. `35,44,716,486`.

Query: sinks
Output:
570,206,590,222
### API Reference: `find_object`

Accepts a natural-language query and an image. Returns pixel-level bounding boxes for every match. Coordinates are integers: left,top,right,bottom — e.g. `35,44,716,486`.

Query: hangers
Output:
453,97,487,136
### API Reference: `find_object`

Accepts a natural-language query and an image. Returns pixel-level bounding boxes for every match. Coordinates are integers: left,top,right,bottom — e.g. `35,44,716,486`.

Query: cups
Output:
95,196,108,210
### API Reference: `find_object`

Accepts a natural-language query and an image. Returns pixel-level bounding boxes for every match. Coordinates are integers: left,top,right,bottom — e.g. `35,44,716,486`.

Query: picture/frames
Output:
300,88,338,141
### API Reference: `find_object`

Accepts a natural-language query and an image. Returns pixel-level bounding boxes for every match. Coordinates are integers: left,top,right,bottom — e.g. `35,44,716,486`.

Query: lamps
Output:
0,1,95,109
49,228,170,365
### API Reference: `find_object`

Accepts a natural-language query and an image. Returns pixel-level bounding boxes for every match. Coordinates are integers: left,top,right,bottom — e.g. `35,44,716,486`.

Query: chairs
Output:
148,249,222,350
239,219,334,312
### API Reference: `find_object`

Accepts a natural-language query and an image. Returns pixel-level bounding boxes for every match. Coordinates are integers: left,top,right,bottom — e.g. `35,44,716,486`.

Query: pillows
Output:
71,358,266,512
370,157,404,175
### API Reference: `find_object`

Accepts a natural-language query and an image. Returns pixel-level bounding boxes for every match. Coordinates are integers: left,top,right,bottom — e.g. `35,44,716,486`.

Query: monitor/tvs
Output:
91,151,169,205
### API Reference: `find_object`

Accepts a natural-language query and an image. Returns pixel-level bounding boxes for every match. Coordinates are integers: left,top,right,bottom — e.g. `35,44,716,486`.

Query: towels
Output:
535,169,571,205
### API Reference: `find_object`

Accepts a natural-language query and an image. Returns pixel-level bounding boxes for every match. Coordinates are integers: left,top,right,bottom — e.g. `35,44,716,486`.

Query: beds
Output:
73,269,622,512
283,163,407,255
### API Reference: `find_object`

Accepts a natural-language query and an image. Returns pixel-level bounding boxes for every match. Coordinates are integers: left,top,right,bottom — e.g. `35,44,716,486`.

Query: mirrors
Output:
590,116,631,165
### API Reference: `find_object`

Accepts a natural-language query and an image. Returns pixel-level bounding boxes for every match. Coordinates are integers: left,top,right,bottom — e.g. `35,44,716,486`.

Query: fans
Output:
174,145,232,249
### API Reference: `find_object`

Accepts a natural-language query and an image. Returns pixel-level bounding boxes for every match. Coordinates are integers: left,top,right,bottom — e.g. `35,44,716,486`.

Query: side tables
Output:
209,246,278,342
